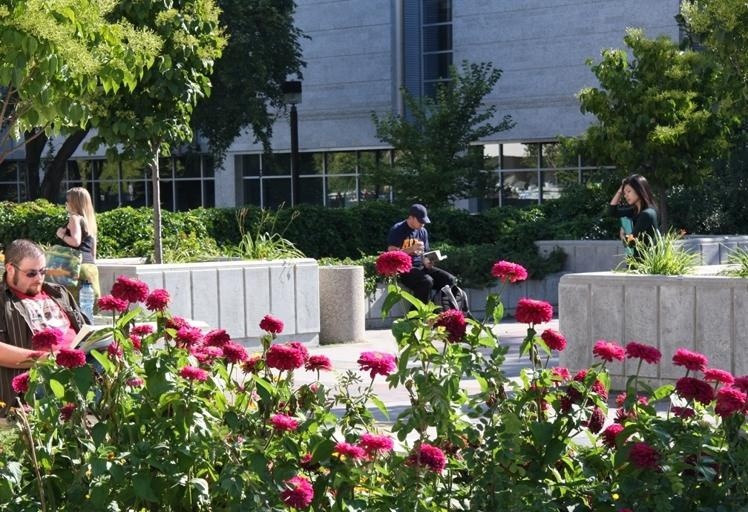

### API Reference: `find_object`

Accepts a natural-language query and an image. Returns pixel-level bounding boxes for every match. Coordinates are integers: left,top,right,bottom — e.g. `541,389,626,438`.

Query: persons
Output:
55,187,101,316
608,174,659,269
0,239,104,422
386,204,459,313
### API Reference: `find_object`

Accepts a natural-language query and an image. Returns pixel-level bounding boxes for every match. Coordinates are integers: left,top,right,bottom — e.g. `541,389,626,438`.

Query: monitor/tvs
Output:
619,216,635,258
424,250,449,264
68,322,157,356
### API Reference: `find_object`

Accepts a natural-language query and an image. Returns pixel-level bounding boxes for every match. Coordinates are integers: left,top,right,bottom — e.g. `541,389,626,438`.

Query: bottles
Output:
410,204,431,224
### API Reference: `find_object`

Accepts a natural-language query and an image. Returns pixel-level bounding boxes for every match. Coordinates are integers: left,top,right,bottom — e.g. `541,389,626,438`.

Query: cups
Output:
10,263,47,277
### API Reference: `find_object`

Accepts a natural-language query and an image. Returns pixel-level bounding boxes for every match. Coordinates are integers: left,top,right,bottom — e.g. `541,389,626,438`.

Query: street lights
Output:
45,244,83,287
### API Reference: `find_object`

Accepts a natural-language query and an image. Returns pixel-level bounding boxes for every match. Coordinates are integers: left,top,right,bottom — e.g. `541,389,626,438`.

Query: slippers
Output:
433,284,470,317
